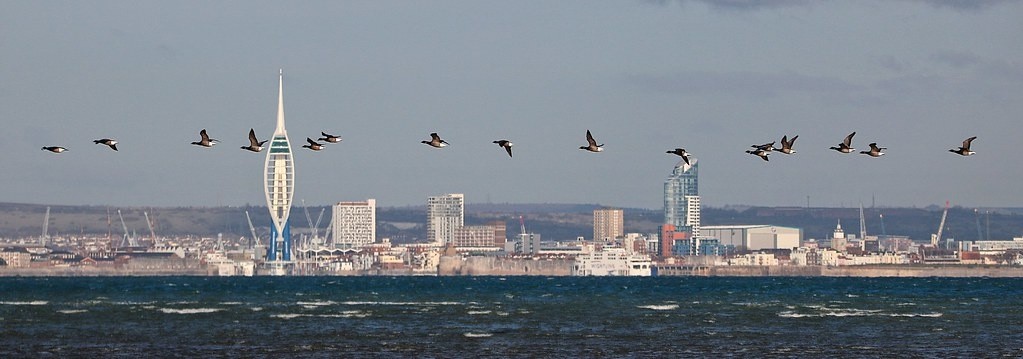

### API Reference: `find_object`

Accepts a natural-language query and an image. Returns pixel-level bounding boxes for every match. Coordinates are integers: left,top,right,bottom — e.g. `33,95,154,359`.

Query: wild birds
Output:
772,135,799,155
316,132,342,143
93,138,119,152
492,140,514,157
666,148,692,166
240,128,269,152
191,129,221,147
579,129,605,152
302,138,326,150
948,136,977,157
829,132,857,153
745,141,777,162
420,132,450,148
40,146,69,153
859,142,887,157
